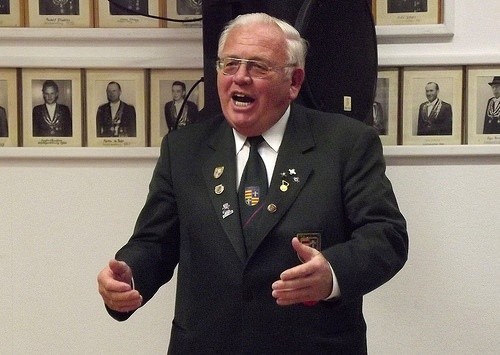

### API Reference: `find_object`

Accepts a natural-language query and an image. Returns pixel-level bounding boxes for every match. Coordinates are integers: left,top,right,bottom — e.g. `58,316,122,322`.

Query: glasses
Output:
216,57,296,78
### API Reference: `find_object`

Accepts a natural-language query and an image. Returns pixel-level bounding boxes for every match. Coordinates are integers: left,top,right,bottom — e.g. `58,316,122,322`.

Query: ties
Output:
238,135,268,231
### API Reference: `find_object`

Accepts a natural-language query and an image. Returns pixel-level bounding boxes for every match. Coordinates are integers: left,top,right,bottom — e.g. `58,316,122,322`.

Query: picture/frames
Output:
94,0,163,27
84,69,148,147
163,0,203,27
0,68,19,147
465,63,500,144
25,0,94,27
373,65,401,145
401,64,465,145
372,0,443,25
148,68,204,147
20,68,84,147
0,0,25,27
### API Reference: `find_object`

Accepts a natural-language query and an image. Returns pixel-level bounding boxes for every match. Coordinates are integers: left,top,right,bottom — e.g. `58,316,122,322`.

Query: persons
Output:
373,98,385,135
165,80,198,133
97,12,410,355
417,81,452,135
483,75,500,134
96,81,137,137
33,80,72,137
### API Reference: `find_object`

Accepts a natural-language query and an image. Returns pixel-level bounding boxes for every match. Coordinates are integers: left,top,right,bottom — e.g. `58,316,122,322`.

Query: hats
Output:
488,76,500,87
